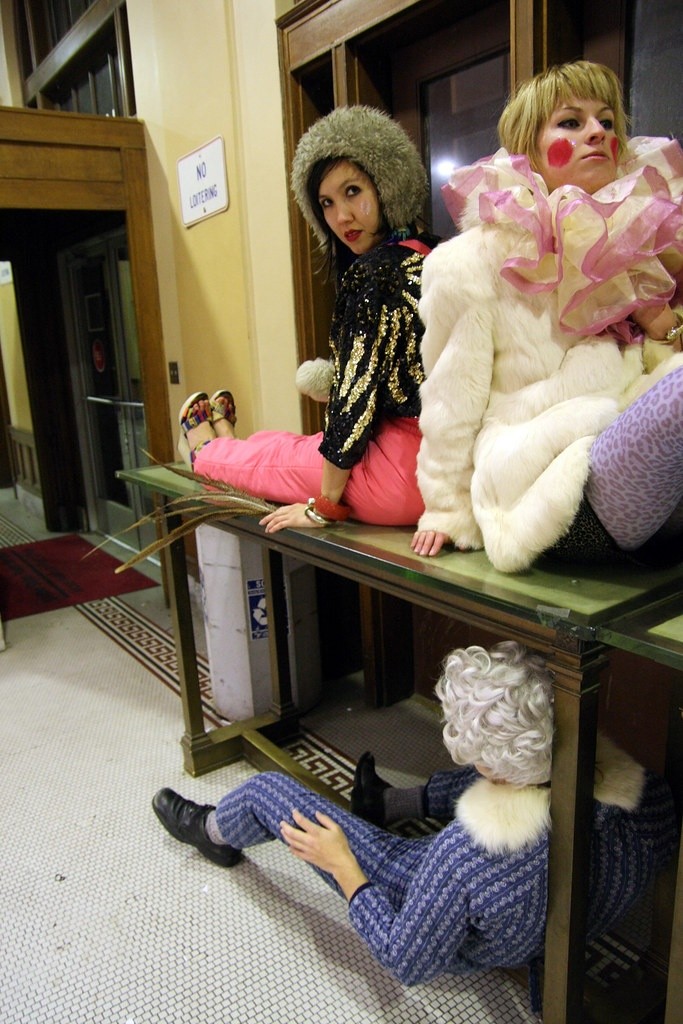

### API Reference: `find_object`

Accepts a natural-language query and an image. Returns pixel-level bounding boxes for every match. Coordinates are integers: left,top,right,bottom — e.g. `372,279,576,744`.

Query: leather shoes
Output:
150,786,242,868
350,750,393,829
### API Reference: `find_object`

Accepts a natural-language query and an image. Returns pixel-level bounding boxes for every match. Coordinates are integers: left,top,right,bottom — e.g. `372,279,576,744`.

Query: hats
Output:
291,105,429,260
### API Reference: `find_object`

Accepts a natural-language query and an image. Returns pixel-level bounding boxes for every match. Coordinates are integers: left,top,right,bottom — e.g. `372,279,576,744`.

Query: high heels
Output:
176,389,236,474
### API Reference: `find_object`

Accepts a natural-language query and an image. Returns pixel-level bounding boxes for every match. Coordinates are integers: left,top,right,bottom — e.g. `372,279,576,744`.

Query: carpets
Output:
0,533,162,621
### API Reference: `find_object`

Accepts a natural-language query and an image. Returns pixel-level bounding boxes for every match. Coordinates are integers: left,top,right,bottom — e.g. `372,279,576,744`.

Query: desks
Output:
113,459,683,1024
